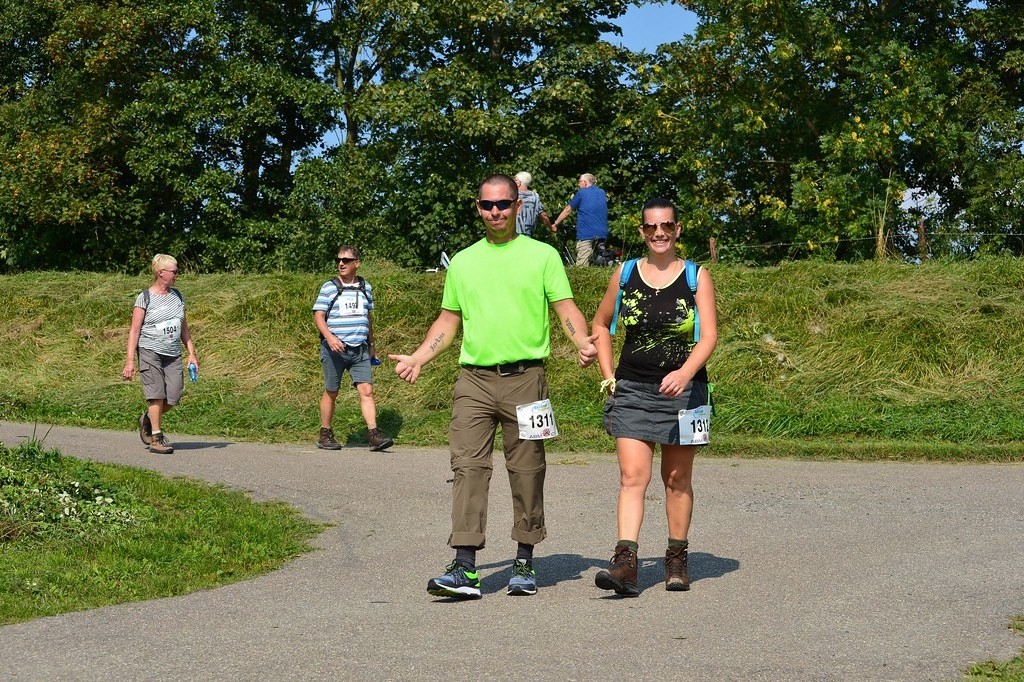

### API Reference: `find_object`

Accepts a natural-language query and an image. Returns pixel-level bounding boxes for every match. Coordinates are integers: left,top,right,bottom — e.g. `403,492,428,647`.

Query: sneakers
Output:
427,558,482,600
506,559,538,595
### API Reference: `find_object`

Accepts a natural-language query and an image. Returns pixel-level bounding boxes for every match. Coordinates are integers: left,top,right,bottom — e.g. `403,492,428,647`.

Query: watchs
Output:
370,342,375,346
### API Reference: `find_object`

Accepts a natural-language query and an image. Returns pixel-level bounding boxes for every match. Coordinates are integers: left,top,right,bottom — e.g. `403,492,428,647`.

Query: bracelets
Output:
188,352,196,355
600,378,616,393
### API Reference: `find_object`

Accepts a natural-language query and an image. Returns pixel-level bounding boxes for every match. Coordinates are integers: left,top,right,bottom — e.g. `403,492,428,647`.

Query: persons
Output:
552,173,607,268
312,245,394,451
387,173,600,599
122,254,199,454
591,199,716,597
512,172,552,238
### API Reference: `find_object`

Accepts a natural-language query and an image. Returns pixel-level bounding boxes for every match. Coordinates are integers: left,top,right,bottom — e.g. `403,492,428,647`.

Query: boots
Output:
317,428,342,450
139,412,153,445
149,433,174,453
664,543,690,590
595,544,639,597
367,428,393,451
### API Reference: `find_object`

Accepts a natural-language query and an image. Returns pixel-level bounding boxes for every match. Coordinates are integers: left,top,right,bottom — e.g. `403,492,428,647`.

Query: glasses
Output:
643,221,677,235
161,269,177,275
479,199,517,211
335,258,358,263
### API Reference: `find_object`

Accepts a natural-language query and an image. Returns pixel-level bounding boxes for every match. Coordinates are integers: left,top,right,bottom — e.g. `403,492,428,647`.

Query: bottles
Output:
371,358,380,365
189,364,197,381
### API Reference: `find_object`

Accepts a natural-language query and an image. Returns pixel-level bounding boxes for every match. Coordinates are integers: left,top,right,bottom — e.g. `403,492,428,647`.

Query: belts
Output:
468,359,543,377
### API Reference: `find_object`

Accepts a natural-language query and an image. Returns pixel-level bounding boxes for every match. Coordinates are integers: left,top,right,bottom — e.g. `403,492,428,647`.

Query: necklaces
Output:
647,256,676,295
